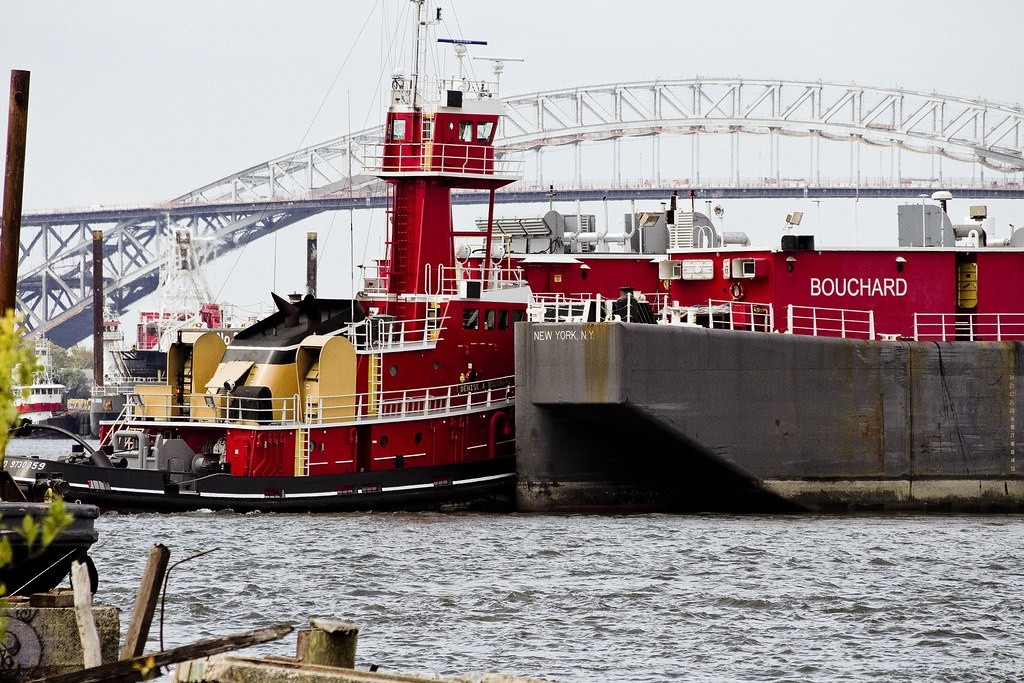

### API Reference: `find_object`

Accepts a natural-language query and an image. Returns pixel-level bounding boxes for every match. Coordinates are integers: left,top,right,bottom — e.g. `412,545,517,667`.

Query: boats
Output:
1,0,537,598
